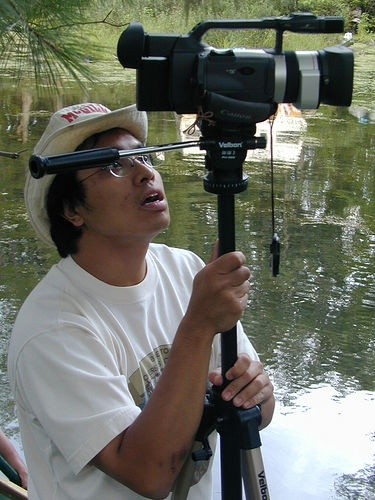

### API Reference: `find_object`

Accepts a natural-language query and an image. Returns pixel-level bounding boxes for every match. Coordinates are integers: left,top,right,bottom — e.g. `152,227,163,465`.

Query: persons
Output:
8,103,276,500
0,428,28,489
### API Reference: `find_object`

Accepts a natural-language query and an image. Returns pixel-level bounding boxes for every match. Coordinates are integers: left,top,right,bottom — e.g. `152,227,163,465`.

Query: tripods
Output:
29,115,270,500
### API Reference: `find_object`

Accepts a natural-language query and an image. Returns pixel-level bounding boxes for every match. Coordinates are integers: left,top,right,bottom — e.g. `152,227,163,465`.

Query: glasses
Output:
79,145,165,183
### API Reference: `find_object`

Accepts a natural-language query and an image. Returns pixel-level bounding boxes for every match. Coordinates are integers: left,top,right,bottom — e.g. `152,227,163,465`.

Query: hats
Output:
24,102,148,247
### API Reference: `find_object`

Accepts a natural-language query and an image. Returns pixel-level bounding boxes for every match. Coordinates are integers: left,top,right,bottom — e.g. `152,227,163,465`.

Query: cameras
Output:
116,12,353,115
